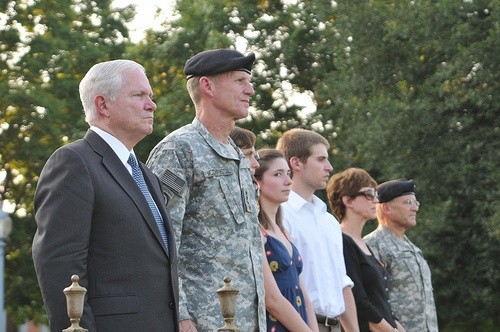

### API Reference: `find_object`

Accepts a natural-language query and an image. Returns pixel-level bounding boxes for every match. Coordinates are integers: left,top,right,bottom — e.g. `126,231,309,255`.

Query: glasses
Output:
398,199,420,210
354,188,378,200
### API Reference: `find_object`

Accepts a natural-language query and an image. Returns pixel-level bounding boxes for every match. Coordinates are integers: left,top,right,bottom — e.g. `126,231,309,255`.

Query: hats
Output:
377,179,416,203
183,49,255,79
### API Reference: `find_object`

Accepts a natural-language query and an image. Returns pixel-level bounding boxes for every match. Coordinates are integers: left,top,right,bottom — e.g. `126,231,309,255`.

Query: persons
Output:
275,128,361,332
145,49,267,332
251,147,320,332
361,177,439,332
227,126,260,182
325,167,406,332
32,60,180,332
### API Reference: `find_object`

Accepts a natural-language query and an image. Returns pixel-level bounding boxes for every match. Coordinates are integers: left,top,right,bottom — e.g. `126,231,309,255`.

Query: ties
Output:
126,152,170,257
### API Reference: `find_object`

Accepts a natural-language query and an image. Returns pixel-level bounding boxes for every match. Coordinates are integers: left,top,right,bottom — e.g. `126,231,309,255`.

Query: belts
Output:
316,314,339,326
